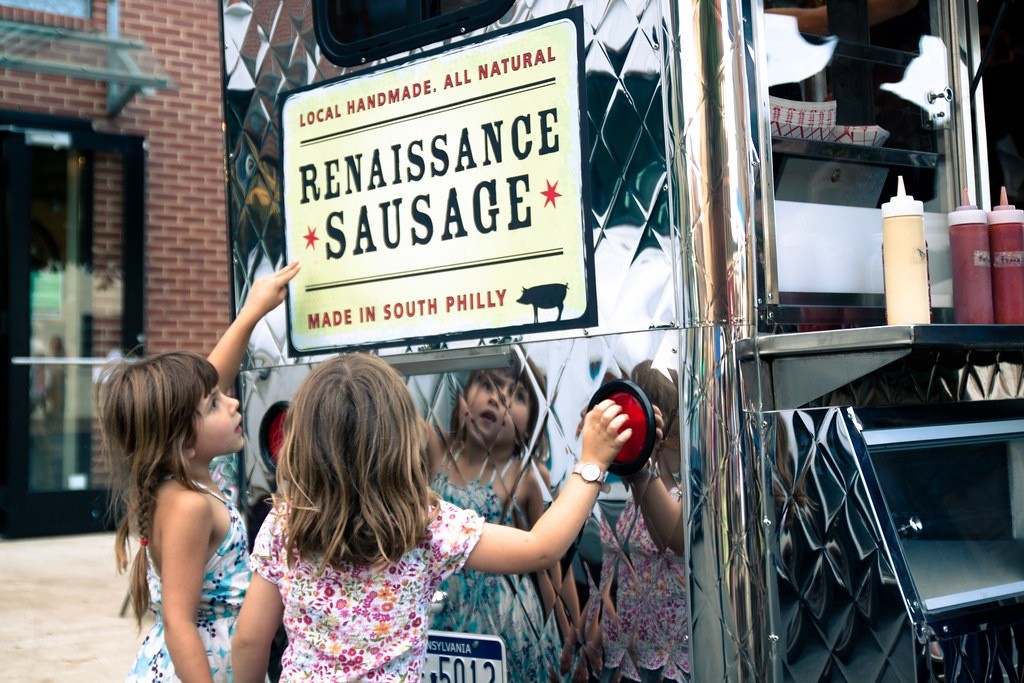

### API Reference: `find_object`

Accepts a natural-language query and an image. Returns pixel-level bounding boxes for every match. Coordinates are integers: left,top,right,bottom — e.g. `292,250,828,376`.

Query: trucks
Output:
221,0,1024,683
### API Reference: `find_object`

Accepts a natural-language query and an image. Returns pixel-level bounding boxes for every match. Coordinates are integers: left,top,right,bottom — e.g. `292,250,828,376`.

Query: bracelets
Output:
622,458,659,492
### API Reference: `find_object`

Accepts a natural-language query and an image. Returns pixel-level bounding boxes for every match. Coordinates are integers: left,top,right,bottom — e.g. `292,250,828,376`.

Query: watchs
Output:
571,462,611,493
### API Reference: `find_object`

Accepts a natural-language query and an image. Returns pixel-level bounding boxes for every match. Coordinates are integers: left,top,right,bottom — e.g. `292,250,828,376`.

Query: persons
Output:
427,350,693,683
769,0,937,208
232,352,633,683
101,260,301,683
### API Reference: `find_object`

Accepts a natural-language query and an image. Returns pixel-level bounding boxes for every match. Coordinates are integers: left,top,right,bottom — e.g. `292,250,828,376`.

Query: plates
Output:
770,95,889,148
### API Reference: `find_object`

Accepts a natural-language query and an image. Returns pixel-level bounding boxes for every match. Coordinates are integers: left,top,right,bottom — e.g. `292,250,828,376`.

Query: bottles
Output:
949,188,994,324
883,175,931,325
987,186,1024,324
